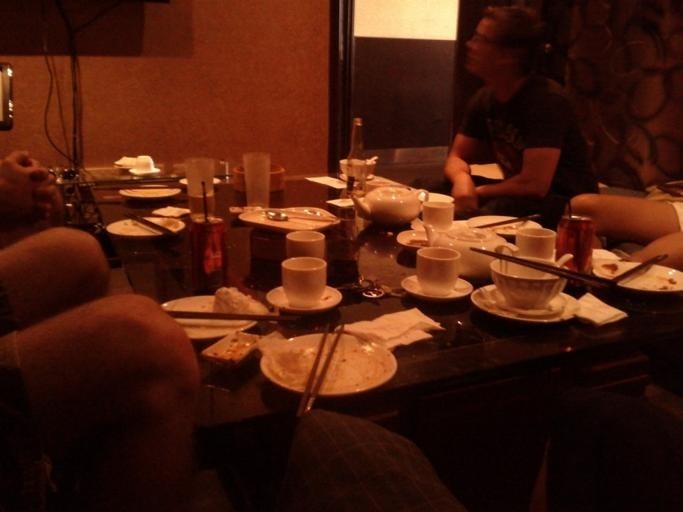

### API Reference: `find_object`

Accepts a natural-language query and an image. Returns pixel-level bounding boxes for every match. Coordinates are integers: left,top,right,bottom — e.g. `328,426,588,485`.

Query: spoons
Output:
230,204,290,224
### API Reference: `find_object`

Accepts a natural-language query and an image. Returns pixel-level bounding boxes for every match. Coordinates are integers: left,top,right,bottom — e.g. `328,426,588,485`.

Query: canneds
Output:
191,217,226,280
556,215,594,276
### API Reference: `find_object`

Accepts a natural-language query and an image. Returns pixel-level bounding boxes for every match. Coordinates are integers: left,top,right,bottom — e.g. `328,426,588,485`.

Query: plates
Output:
164,293,267,339
267,284,342,312
119,184,182,201
337,172,375,182
471,281,580,324
400,275,472,302
239,204,342,234
113,158,135,170
589,255,683,296
179,177,221,187
105,217,186,240
465,214,543,241
396,227,437,251
128,168,161,177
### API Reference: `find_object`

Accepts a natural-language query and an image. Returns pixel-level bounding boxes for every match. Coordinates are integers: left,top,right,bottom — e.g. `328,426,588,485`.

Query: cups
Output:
517,226,556,258
285,228,325,260
279,257,329,304
422,198,457,232
135,156,154,172
244,151,271,209
184,158,215,223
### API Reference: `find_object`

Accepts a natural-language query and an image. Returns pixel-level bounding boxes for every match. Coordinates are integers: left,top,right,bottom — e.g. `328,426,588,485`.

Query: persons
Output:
439,3,603,235
0,148,65,253
556,191,682,273
0,223,204,511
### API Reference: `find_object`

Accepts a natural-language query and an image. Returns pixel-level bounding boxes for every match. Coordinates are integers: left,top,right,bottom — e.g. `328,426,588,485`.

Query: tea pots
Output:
354,184,430,225
423,221,518,280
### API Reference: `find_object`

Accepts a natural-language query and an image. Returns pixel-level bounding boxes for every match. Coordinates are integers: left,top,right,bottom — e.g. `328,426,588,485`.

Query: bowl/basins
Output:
230,165,285,194
338,158,376,178
490,250,568,313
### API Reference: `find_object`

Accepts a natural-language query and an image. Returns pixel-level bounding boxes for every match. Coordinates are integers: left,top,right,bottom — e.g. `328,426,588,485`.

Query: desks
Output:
50,159,235,219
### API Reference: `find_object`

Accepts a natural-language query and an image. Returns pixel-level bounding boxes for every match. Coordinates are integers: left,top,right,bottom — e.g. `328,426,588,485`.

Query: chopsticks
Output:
120,186,181,190
166,307,296,326
606,252,667,290
296,321,344,417
473,212,541,229
123,212,179,237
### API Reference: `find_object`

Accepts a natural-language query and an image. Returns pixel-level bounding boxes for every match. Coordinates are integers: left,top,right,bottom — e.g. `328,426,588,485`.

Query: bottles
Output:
345,118,368,198
